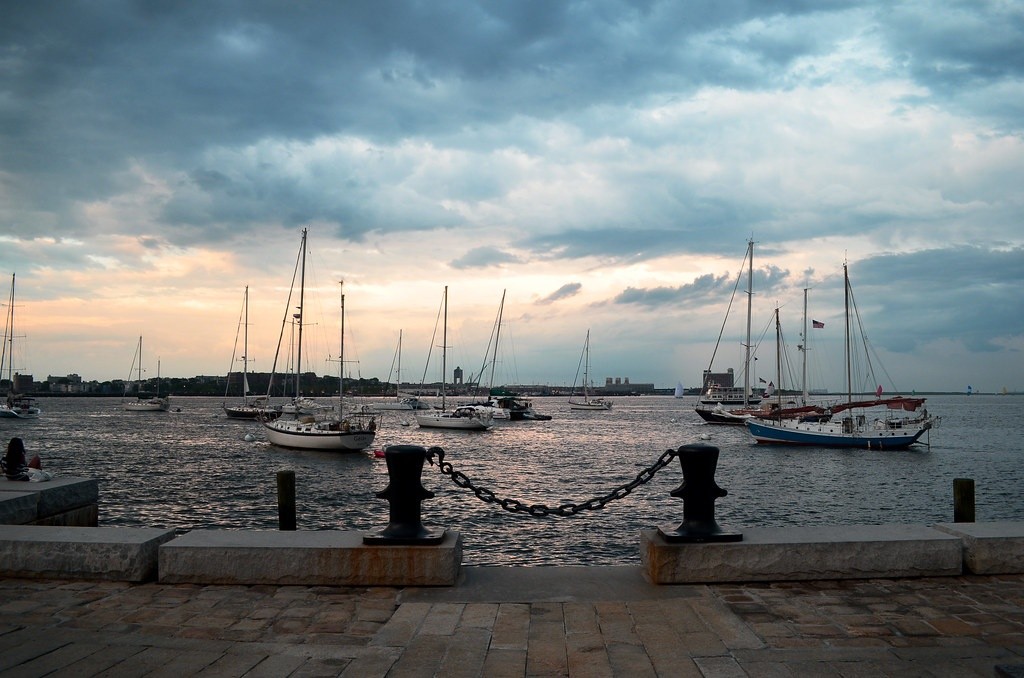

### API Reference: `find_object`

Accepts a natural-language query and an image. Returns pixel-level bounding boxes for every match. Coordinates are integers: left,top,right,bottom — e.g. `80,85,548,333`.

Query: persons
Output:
4,437,43,481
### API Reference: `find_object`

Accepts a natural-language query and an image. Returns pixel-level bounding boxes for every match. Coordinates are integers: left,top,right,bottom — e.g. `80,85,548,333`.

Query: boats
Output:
700,385,763,405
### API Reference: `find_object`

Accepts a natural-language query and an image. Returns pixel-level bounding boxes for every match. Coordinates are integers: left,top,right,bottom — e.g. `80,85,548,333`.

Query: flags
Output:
759,378,766,384
812,320,826,329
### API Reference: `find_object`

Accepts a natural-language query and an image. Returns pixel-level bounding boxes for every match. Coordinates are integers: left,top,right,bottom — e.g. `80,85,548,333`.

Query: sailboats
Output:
255,227,378,453
411,285,496,432
0,270,42,420
219,285,283,422
674,381,684,399
120,335,171,411
743,264,942,452
268,319,335,415
694,240,834,428
456,289,531,421
372,328,430,411
567,327,614,411
346,369,380,417
